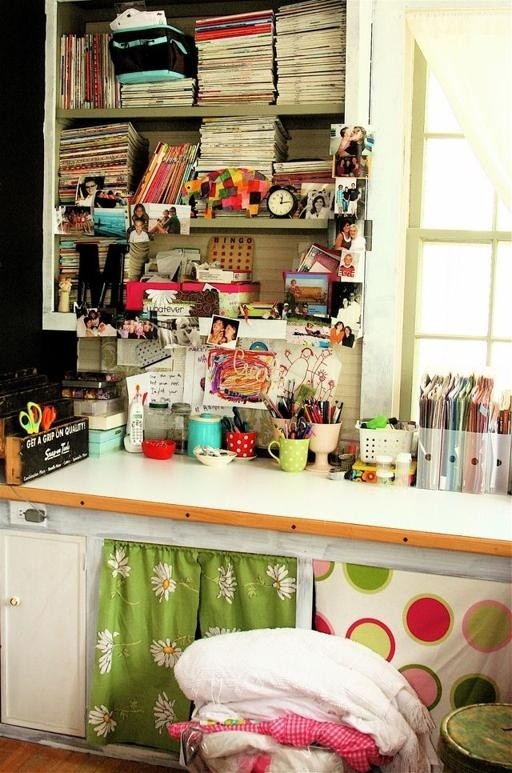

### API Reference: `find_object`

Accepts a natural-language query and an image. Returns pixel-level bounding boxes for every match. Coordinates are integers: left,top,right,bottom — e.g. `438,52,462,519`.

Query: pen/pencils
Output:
274,419,314,439
297,396,344,424
223,406,249,433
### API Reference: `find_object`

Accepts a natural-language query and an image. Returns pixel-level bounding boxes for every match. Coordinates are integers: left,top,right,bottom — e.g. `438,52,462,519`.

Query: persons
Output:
338,253,356,278
334,220,366,253
342,183,361,217
77,309,116,338
335,126,367,176
331,320,355,349
208,320,237,351
305,321,326,341
162,318,201,349
117,315,158,339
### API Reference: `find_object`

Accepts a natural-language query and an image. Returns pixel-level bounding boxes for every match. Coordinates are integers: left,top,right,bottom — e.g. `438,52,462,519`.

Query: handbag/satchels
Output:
108,25,184,82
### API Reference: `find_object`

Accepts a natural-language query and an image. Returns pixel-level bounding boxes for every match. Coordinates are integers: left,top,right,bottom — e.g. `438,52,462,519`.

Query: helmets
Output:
109,8,167,30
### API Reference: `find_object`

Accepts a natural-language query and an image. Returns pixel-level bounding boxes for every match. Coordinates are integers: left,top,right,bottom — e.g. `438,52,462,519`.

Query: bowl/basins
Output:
198,449,236,465
143,439,176,459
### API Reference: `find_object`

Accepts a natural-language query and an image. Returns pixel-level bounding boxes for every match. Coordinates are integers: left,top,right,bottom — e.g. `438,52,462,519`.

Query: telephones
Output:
123,400,146,453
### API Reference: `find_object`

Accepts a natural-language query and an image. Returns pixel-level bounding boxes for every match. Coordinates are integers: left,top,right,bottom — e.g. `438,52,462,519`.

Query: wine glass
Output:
303,418,342,471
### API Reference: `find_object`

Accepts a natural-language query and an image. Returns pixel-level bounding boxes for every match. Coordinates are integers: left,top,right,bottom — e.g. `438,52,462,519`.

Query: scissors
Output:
19,401,56,436
221,419,234,433
289,416,306,438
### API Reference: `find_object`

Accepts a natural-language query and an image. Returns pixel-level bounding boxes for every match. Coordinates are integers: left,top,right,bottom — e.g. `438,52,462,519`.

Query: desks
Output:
0,438,511,772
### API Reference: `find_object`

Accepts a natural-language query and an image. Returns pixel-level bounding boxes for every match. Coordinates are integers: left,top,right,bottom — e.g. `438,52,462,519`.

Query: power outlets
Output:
5,501,48,526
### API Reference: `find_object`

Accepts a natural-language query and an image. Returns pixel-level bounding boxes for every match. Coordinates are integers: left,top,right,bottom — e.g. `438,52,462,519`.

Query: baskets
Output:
355,424,417,464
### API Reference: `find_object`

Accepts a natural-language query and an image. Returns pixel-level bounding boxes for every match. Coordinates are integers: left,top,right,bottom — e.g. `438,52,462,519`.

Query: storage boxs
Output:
122,277,262,320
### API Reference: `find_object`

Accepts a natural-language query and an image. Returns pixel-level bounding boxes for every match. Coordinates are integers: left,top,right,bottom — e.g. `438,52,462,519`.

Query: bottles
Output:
168,403,190,453
188,413,223,456
374,450,412,488
144,401,171,439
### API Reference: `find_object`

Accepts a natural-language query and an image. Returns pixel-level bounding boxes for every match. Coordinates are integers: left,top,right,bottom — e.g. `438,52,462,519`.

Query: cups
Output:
267,438,309,472
225,430,257,457
272,417,291,440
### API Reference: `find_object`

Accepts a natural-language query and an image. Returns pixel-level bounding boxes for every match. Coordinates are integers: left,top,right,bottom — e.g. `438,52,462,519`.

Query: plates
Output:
232,454,256,461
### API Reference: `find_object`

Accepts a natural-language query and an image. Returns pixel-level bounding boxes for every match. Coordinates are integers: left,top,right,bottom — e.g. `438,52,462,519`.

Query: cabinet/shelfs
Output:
35,0,378,345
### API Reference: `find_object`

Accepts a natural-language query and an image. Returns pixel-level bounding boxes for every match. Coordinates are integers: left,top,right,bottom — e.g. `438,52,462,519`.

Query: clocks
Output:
261,179,300,221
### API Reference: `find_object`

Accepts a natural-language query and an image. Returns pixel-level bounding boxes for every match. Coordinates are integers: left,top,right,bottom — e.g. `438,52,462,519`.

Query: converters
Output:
25,509,45,523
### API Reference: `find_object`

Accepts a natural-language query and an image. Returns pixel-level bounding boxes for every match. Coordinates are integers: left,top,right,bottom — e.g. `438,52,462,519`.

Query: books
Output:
498,389,512,433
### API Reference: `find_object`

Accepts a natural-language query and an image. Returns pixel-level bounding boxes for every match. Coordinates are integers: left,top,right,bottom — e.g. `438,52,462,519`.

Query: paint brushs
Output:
261,377,295,419
